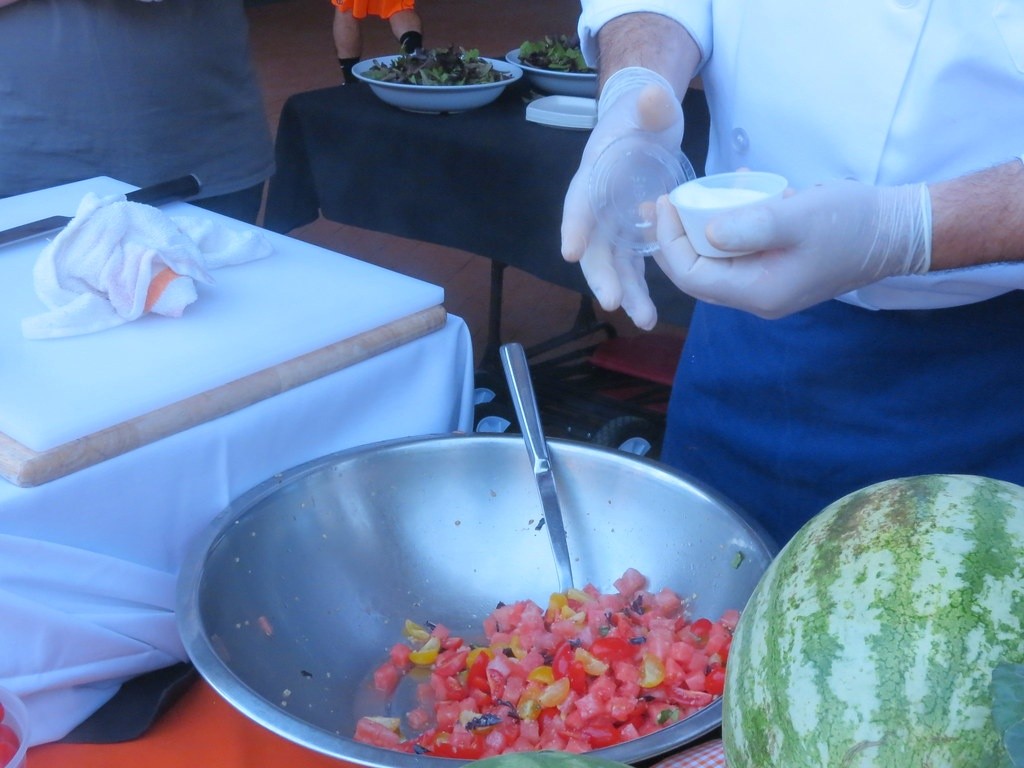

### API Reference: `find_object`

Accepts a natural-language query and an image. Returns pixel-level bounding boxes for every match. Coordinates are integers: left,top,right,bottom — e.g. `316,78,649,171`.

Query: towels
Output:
23,192,274,341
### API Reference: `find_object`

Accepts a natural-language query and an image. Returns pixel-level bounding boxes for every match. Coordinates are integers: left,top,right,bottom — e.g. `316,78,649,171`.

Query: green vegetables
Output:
359,36,515,86
517,31,597,75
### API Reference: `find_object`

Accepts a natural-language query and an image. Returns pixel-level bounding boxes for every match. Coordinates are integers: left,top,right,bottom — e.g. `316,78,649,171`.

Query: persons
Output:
561,0,1024,555
331,0,422,86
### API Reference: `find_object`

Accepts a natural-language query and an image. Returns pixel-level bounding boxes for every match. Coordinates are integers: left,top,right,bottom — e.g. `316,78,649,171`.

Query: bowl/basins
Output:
351,53,524,115
506,49,601,98
669,172,787,257
174,431,782,768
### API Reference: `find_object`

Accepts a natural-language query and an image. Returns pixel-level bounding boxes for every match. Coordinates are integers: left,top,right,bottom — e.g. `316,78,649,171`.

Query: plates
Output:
525,93,598,129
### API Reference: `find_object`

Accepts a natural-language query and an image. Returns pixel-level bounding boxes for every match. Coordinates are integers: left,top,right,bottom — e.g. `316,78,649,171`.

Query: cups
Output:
0,687,33,768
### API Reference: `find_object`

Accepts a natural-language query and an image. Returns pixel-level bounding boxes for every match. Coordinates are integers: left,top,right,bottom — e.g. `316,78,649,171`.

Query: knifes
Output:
0,165,269,248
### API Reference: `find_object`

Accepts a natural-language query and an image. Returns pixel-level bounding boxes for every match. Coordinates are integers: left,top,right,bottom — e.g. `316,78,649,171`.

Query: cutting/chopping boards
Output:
0,174,444,452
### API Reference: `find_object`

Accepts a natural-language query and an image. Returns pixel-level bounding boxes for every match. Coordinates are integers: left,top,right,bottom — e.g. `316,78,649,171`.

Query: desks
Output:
262,56,711,455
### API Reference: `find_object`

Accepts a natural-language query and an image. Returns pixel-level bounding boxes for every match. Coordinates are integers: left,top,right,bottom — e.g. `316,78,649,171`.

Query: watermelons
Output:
721,473,1024,768
353,567,741,757
